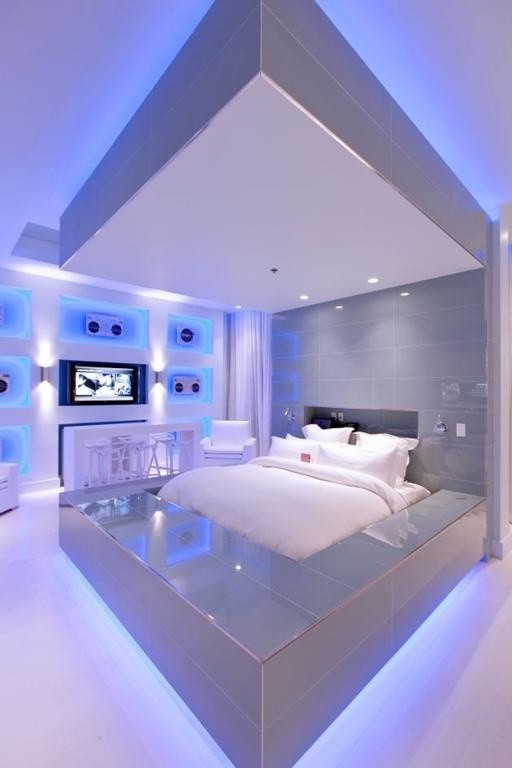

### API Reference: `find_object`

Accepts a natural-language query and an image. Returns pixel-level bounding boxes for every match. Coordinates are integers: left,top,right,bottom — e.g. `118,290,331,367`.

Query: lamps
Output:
433,413,447,433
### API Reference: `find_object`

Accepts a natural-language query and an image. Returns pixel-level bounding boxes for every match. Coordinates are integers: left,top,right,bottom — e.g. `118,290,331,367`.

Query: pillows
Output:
268,424,419,489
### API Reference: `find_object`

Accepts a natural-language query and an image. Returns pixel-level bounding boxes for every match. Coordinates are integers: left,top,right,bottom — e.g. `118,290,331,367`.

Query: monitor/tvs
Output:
72,361,138,404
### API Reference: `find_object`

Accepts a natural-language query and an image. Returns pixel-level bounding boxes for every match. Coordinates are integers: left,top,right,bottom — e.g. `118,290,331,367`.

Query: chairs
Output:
200,419,257,464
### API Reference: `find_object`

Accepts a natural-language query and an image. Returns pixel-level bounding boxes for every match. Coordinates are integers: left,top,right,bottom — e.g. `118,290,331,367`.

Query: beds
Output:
155,432,433,562
